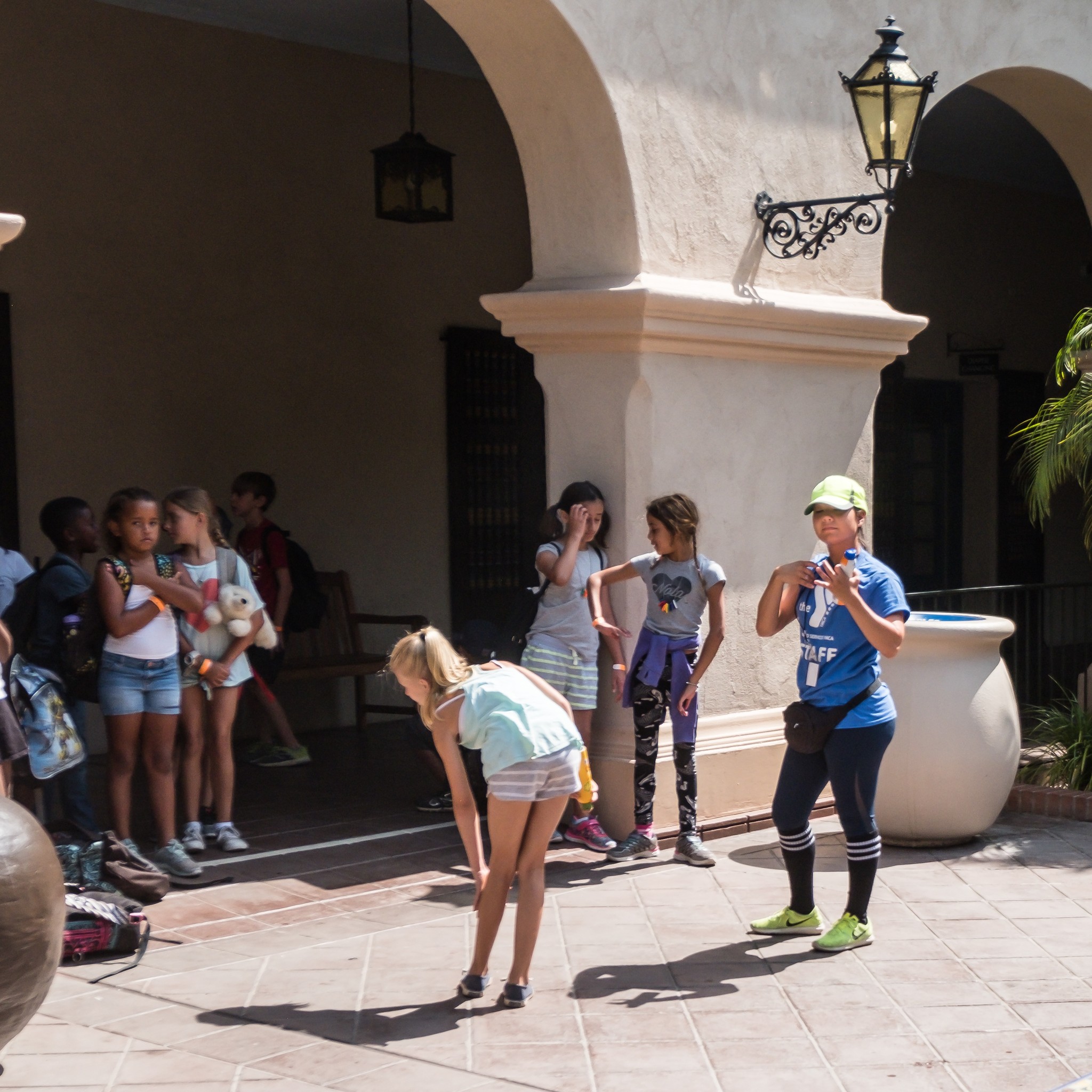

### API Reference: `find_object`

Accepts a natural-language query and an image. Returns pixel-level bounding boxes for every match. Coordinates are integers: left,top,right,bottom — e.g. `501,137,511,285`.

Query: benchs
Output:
269,570,431,740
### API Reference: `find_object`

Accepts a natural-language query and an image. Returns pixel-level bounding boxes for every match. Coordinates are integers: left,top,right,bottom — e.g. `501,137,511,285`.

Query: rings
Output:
805,575,809,581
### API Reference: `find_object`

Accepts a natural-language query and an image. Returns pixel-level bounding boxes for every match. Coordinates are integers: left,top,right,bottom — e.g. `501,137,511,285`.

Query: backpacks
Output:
2,556,88,656
510,541,564,647
259,524,324,631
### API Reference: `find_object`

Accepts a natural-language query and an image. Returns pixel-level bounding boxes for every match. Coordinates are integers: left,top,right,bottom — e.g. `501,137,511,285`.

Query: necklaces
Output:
658,546,694,613
575,543,591,598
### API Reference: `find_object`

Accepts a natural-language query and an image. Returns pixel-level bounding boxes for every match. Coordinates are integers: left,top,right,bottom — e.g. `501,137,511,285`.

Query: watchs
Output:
183,650,202,668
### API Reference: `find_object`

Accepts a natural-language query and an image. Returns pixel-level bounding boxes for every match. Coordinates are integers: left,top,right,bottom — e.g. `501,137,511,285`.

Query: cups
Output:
62,615,82,640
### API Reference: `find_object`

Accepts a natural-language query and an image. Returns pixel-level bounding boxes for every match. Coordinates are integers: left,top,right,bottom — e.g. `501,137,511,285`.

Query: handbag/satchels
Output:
782,679,881,754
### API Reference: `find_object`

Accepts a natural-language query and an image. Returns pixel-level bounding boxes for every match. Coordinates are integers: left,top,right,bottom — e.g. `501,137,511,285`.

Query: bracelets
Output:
147,595,166,612
612,664,627,672
592,617,607,628
273,625,283,632
198,658,214,675
686,681,700,686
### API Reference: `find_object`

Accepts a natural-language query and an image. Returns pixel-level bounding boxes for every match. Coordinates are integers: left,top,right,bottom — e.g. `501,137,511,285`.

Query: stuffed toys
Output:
203,582,278,650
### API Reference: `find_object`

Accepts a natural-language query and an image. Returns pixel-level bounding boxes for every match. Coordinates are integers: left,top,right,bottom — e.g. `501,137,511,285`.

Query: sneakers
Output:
605,829,659,861
251,745,311,767
499,975,535,1007
750,901,825,935
672,833,717,866
184,820,206,852
150,837,204,878
119,838,144,858
812,912,875,952
211,821,250,852
563,812,617,852
460,968,494,997
201,822,217,837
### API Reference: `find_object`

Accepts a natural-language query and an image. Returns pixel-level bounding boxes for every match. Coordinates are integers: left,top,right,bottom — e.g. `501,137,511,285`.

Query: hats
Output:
804,476,869,515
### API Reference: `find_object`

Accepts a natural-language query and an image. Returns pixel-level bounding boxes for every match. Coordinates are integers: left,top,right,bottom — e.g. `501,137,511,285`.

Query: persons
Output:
92,488,207,879
227,468,314,770
0,495,40,802
750,475,911,956
411,620,518,815
385,626,585,1010
518,480,631,853
30,494,110,844
585,494,728,870
161,486,265,853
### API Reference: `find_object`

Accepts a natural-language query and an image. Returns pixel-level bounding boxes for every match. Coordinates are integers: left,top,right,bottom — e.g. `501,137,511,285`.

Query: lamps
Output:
750,13,936,264
366,1,457,226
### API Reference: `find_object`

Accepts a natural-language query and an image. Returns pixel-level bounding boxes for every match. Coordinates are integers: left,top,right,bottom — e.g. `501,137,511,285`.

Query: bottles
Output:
832,548,857,606
